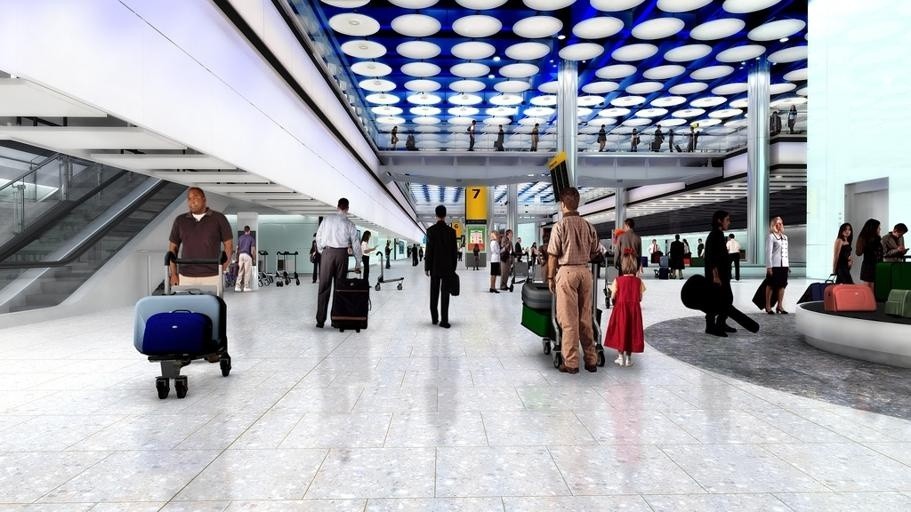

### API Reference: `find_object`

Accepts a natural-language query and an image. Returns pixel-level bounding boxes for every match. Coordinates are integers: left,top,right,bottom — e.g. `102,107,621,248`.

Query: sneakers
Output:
432,320,451,328
235,287,252,291
316,322,324,328
705,321,736,336
614,357,634,367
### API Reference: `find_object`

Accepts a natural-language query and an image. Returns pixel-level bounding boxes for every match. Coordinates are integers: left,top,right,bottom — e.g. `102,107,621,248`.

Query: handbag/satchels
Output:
142,310,212,355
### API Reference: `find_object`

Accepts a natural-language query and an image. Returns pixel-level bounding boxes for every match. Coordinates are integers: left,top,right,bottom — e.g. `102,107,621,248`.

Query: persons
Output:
385,242,393,269
530,124,540,152
488,230,510,293
704,211,738,338
391,126,399,150
497,125,505,151
546,187,600,375
631,128,638,152
765,216,790,315
599,124,607,152
424,204,457,328
548,277,553,279
361,230,377,288
314,198,363,328
771,111,782,135
606,250,644,367
859,218,884,289
408,217,705,279
468,120,477,150
169,186,233,366
788,105,798,134
501,230,513,290
726,234,742,281
406,134,416,151
310,233,321,284
653,124,701,153
879,223,908,262
235,226,257,293
833,223,855,283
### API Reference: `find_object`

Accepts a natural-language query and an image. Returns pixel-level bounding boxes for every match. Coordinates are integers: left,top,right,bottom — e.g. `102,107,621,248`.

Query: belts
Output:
323,246,348,250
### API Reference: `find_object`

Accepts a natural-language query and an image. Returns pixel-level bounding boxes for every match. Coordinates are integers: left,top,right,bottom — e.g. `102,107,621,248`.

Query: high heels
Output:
766,308,788,314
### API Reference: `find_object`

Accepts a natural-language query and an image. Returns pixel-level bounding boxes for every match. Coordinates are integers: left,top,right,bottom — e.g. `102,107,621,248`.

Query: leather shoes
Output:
558,364,597,374
490,287,509,293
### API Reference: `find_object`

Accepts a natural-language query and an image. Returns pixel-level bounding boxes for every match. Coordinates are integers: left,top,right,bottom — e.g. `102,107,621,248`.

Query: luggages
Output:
133,291,227,356
659,256,668,279
752,278,788,307
797,261,911,317
675,145,682,152
330,278,371,333
521,282,602,338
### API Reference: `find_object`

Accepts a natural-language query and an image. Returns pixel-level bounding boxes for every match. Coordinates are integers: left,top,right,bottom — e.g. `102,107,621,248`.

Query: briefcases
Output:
450,272,460,296
